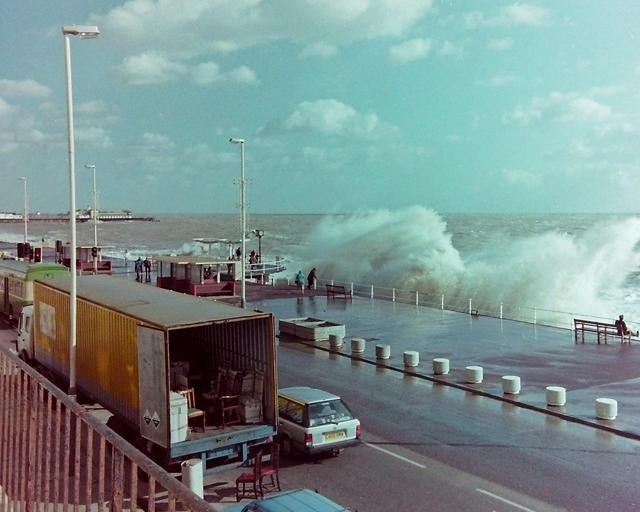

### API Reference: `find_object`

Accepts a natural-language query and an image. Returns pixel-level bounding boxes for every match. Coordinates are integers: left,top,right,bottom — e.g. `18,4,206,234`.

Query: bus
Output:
1,260,70,324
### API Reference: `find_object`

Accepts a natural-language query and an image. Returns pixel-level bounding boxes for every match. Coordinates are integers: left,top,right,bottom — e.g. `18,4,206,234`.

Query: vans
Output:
220,488,358,512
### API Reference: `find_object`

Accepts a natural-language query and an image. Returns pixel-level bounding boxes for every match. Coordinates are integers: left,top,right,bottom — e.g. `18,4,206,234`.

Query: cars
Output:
278,387,363,457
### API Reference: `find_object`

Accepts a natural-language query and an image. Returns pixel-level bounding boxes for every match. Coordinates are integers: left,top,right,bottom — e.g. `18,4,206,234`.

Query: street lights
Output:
20,177,28,245
84,163,97,276
229,138,246,307
60,24,102,399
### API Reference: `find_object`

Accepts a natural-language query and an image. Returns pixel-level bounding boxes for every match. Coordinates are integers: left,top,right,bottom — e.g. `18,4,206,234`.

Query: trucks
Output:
18,275,279,480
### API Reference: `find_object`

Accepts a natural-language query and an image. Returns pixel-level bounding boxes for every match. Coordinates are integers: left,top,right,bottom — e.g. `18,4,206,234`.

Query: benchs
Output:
574,319,631,345
326,284,352,299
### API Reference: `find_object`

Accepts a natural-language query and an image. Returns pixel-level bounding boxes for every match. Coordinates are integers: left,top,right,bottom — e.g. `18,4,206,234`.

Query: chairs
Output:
236,449,263,502
261,443,281,492
200,367,243,429
178,387,206,433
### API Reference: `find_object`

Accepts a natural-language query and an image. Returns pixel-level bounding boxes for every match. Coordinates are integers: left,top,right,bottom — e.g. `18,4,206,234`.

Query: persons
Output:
615,314,639,337
123,249,130,266
133,254,152,282
248,250,259,266
232,255,235,259
227,257,232,274
204,266,212,278
296,270,305,290
236,247,240,258
307,267,317,290
